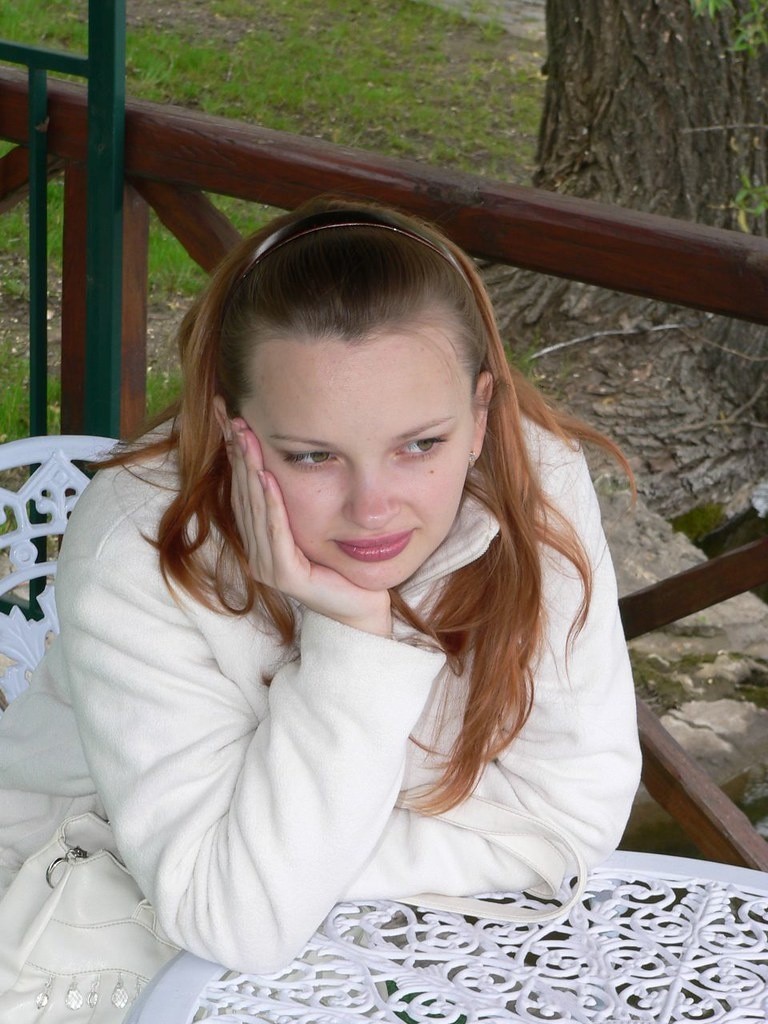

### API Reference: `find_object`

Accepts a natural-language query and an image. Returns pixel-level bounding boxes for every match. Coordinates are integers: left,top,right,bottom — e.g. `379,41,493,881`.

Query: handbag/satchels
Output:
0,810,181,1024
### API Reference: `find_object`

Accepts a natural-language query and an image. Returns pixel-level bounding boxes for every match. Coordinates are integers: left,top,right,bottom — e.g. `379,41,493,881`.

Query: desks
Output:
123,852,768,1024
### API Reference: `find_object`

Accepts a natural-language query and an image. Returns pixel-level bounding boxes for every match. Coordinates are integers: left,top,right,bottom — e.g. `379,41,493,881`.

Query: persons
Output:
0,198,642,1023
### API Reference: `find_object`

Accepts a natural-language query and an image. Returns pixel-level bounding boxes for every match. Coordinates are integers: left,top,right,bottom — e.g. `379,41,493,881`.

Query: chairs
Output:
0,435,130,724
